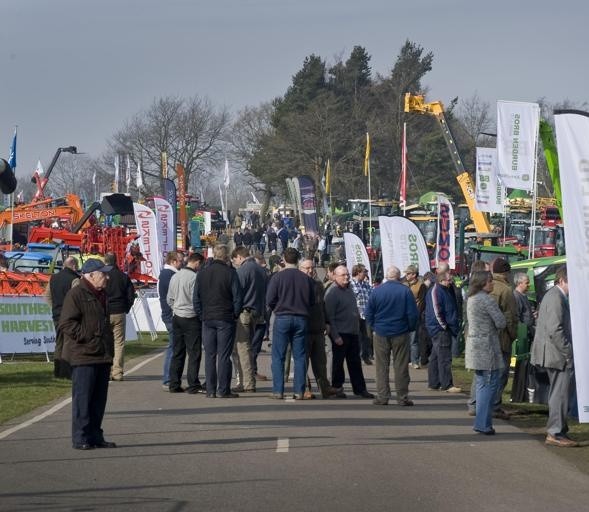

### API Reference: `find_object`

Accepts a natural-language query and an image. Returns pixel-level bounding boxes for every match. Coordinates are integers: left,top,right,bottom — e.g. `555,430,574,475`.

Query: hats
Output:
403,264,419,275
491,257,512,275
81,258,113,274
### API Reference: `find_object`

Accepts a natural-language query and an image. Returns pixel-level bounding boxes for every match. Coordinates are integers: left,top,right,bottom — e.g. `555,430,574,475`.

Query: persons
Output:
50,235,580,448
233,213,299,255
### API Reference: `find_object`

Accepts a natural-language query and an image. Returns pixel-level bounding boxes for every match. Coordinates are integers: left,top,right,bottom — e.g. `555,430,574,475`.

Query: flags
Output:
136,162,143,189
399,127,407,208
191,221,200,247
7,133,17,169
113,156,119,192
125,159,130,186
326,159,331,194
224,160,230,191
133,198,174,279
343,214,430,285
365,134,370,177
36,161,44,177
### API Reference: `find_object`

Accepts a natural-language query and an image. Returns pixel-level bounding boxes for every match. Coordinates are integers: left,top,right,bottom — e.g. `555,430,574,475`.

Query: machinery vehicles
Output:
0,143,227,296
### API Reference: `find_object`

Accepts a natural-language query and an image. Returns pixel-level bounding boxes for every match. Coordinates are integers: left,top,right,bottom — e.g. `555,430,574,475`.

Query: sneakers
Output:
71,437,117,451
353,389,375,398
440,386,464,394
371,398,389,406
161,373,267,398
319,383,347,400
269,392,284,400
397,400,415,407
467,407,509,421
544,433,580,447
472,427,497,436
291,389,316,400
